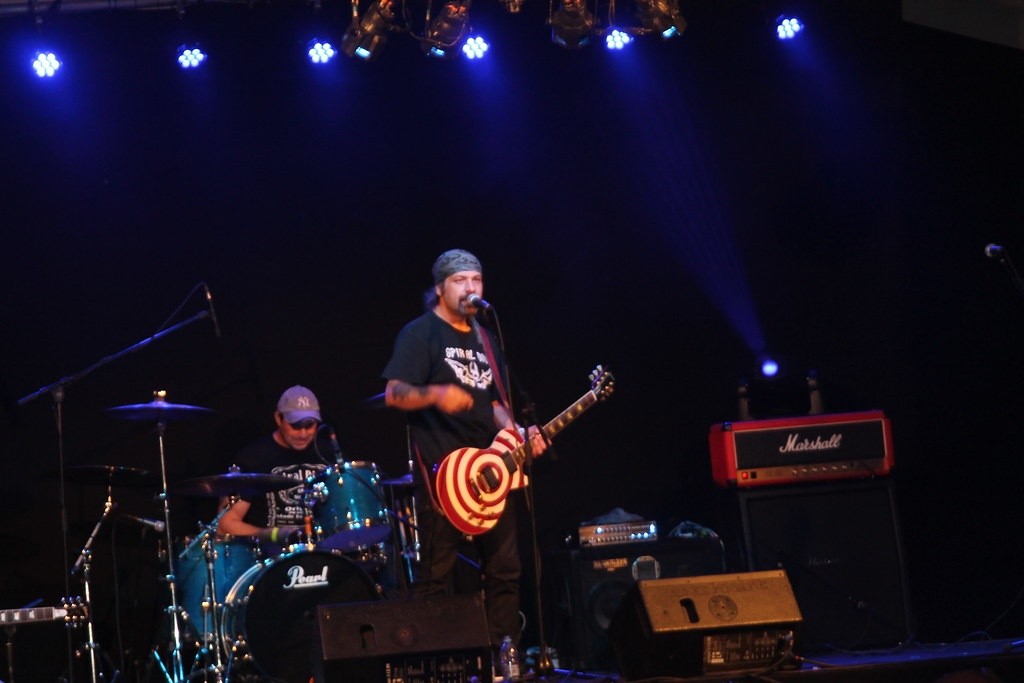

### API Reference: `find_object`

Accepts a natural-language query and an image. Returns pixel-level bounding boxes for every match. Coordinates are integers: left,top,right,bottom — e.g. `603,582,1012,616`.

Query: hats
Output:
278,386,322,424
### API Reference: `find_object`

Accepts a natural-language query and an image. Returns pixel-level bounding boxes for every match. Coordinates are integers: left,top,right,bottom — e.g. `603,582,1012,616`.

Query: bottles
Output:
806,370,823,414
499,635,520,680
737,386,751,420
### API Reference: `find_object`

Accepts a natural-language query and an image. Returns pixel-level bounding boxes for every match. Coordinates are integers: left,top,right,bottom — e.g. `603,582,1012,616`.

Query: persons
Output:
381,249,553,672
217,386,341,558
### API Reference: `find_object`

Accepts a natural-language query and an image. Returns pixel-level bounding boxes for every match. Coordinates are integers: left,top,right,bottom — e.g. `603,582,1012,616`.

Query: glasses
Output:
290,420,317,430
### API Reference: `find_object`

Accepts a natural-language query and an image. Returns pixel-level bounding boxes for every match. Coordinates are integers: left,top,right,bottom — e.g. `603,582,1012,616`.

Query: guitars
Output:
430,364,617,540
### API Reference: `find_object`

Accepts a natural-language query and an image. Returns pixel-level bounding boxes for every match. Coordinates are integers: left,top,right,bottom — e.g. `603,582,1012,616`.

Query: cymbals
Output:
105,400,213,423
179,469,302,497
65,464,152,480
381,473,414,491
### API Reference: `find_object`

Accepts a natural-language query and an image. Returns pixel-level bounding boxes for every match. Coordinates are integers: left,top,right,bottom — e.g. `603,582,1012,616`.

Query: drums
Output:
307,459,395,553
171,536,386,682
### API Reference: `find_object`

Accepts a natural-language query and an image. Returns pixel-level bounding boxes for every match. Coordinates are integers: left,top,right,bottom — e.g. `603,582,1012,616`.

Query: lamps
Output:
634,0,687,39
338,0,401,61
543,0,597,52
419,0,472,64
